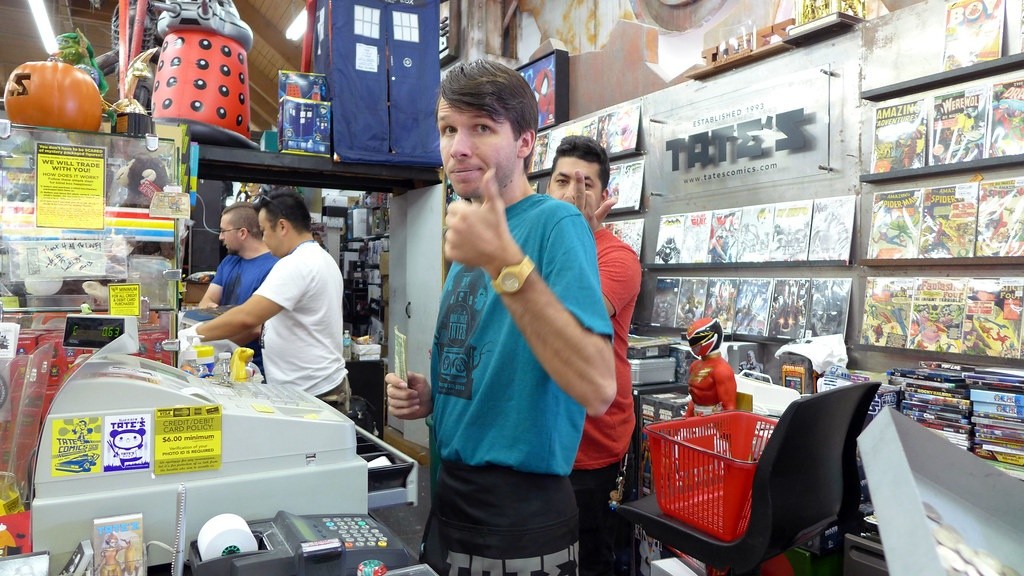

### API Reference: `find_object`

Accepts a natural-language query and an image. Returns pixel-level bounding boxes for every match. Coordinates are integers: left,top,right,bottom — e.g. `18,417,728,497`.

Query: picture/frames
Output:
514,49,569,133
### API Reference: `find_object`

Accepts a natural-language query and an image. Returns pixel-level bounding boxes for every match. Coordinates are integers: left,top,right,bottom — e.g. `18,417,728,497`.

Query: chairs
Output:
613,383,881,576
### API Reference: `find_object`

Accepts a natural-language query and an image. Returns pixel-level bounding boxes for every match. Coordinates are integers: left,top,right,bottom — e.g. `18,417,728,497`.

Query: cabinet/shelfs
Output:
526,51,1024,369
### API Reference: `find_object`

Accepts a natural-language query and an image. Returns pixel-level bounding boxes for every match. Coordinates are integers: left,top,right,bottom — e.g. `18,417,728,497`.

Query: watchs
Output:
491,255,535,295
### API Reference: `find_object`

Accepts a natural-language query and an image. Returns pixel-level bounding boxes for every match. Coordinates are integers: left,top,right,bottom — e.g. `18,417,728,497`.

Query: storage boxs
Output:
322,195,348,225
643,411,777,543
626,358,675,385
380,251,389,340
278,70,331,157
338,242,368,289
347,208,367,238
351,343,380,360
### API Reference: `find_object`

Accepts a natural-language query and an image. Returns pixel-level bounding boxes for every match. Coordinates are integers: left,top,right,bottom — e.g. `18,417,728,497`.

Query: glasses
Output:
259,194,288,217
220,227,250,234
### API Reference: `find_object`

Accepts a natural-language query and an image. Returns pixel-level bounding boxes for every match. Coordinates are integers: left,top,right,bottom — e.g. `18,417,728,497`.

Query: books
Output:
887,362,1024,482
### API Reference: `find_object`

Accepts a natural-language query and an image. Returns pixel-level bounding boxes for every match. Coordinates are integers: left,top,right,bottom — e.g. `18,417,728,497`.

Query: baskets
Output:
642,410,779,544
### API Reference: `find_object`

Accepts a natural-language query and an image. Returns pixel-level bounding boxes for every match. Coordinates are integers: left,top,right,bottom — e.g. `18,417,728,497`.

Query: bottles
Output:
180,337,265,384
343,330,351,359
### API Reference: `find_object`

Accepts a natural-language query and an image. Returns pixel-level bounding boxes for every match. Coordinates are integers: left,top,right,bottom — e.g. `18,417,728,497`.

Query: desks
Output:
345,360,384,440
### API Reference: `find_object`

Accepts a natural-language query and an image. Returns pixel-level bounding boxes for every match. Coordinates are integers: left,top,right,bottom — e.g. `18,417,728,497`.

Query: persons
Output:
385,59,617,576
178,191,348,414
546,135,641,576
685,318,736,437
199,202,279,385
